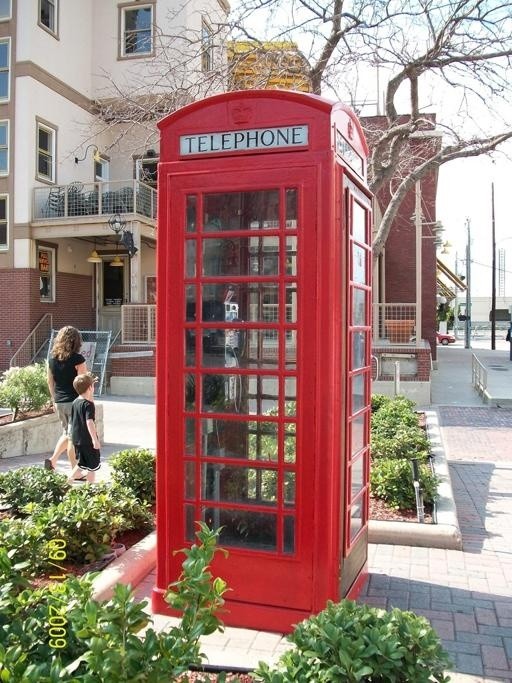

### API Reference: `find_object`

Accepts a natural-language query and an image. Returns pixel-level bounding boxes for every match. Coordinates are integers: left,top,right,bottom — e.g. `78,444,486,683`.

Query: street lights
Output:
464,216,474,348
412,211,447,347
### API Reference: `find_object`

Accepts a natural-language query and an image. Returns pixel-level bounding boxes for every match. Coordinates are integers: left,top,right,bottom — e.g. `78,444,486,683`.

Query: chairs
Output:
45,181,155,217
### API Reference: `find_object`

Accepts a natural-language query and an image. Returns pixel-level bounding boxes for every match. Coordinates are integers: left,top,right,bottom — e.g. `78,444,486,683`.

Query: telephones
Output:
185,300,240,401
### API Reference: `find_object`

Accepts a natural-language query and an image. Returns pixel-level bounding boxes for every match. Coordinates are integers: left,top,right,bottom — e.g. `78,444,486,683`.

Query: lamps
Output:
85,237,102,263
109,240,125,267
74,143,100,163
107,211,139,258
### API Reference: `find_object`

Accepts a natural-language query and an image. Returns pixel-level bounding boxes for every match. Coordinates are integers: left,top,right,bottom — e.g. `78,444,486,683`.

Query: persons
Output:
42,323,89,473
65,369,101,491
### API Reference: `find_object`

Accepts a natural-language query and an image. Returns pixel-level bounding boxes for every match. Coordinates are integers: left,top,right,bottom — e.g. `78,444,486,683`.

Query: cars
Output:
436,330,456,345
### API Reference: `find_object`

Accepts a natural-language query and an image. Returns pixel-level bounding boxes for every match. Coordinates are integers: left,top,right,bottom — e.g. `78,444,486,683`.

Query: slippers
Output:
75,474,87,481
44,459,56,474
